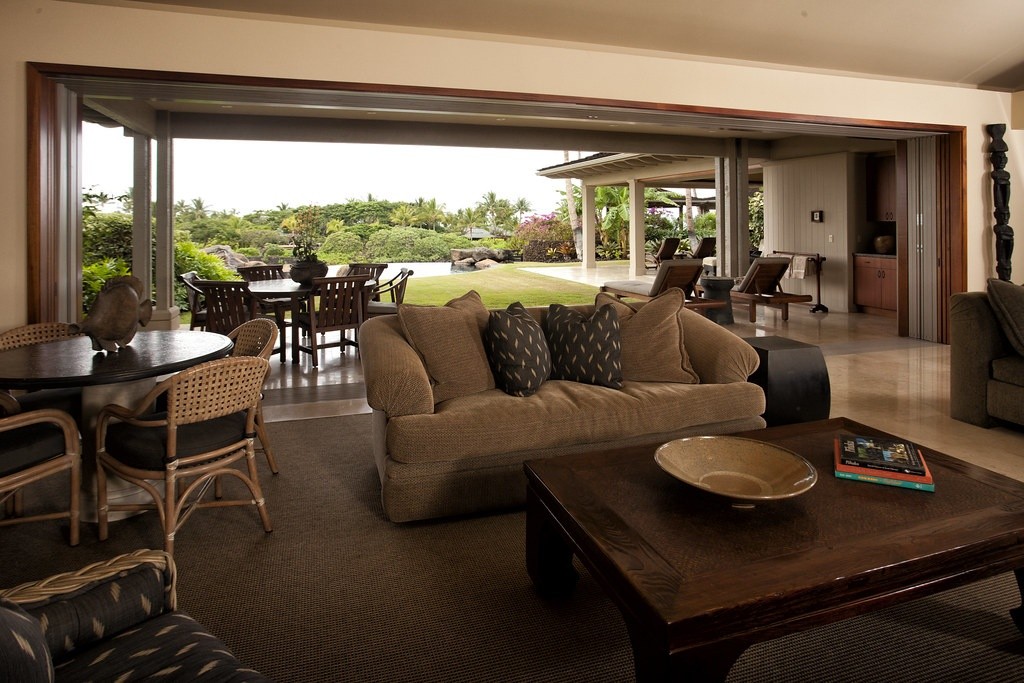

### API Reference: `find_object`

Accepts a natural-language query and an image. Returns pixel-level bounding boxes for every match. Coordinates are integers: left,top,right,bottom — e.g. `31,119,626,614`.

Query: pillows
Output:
397,287,700,405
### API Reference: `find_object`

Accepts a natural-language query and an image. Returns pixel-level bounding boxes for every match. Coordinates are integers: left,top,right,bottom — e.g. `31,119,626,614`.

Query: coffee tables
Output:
522,417,1024,683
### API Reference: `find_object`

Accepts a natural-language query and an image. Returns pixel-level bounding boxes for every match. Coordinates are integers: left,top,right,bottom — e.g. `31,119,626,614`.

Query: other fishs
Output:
68,275,153,353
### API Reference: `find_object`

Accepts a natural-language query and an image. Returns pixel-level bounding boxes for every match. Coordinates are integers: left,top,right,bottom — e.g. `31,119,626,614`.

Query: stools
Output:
702,257,717,276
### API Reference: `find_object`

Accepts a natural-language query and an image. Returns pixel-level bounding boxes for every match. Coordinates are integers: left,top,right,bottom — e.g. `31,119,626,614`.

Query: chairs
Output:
1,262,414,683
599,238,812,323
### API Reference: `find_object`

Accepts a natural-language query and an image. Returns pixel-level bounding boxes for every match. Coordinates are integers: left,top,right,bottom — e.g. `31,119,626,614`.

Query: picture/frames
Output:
810,210,823,222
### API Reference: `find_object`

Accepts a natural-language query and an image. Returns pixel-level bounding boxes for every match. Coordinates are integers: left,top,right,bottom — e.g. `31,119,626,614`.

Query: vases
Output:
290,261,328,284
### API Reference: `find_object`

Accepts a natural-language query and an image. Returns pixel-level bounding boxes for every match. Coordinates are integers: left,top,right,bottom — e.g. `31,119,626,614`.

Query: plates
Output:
654,435,817,510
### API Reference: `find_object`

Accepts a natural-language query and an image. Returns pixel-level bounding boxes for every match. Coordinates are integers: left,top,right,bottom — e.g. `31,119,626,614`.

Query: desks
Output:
675,254,687,259
700,277,735,325
0,329,234,522
232,279,377,364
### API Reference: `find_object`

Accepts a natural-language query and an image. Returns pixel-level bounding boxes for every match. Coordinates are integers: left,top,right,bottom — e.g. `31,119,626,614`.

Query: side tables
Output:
742,337,831,426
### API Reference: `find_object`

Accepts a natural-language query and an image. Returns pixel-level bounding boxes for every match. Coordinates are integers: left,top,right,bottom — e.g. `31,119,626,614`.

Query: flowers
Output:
292,206,320,262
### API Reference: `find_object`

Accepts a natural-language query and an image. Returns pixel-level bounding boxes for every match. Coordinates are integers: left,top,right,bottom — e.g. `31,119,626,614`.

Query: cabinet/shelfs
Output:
866,156,897,221
852,253,897,318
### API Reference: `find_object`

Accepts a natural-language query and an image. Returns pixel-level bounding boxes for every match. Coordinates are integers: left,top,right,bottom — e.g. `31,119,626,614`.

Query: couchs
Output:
949,278,1024,429
358,301,767,523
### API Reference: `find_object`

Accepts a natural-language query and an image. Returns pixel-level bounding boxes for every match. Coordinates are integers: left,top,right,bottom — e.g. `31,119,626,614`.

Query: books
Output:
833,433,935,491
